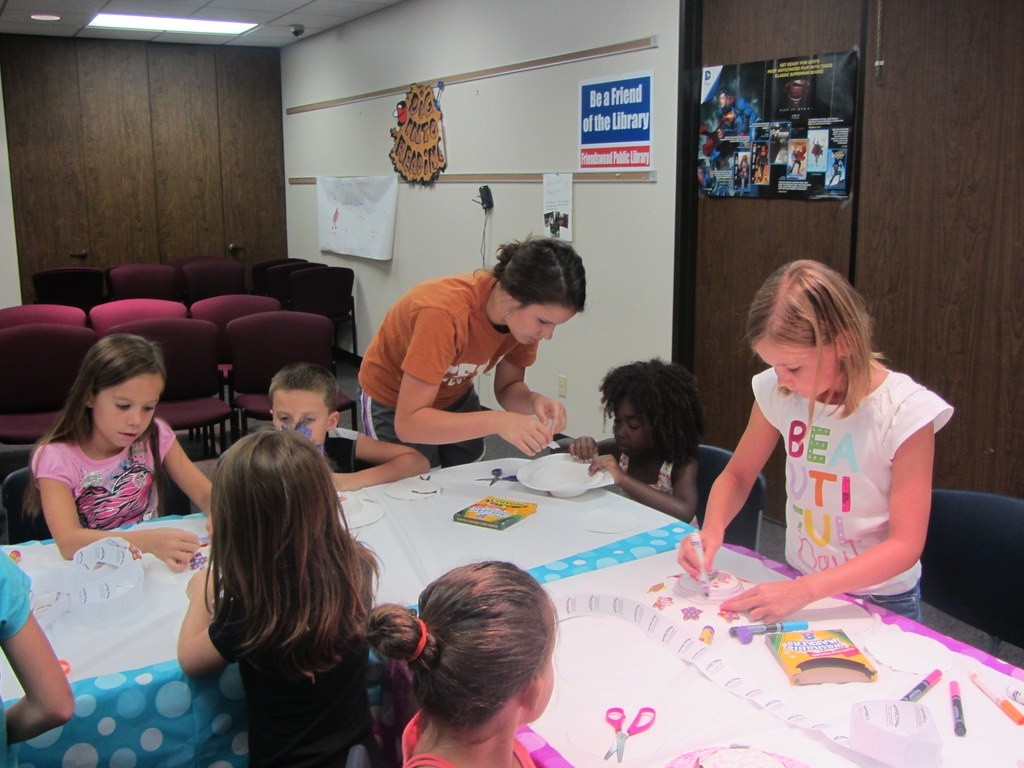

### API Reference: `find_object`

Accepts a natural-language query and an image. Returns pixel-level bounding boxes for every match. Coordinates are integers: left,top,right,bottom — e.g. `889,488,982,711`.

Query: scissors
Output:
604,707,656,762
475,468,519,487
60,660,71,674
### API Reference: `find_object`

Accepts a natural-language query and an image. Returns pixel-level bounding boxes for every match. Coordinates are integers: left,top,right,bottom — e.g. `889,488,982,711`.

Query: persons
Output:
696,87,843,188
356,235,587,470
677,258,956,624
268,363,431,493
21,333,214,573
176,429,381,768
569,360,703,531
365,561,560,768
0,546,75,768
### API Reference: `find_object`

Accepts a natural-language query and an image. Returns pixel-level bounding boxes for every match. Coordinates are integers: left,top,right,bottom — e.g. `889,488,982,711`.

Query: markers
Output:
728,620,809,639
9,550,21,561
1003,687,1023,703
691,534,710,598
900,669,942,701
949,680,967,737
969,671,1024,727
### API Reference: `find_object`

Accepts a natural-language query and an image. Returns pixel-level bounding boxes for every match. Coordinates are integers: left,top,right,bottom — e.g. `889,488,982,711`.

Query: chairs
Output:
918,488,1024,650
0,258,358,547
694,443,767,552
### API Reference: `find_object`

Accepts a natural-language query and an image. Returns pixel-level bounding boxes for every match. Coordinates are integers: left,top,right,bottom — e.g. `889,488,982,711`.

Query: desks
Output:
0,457,1024,768
